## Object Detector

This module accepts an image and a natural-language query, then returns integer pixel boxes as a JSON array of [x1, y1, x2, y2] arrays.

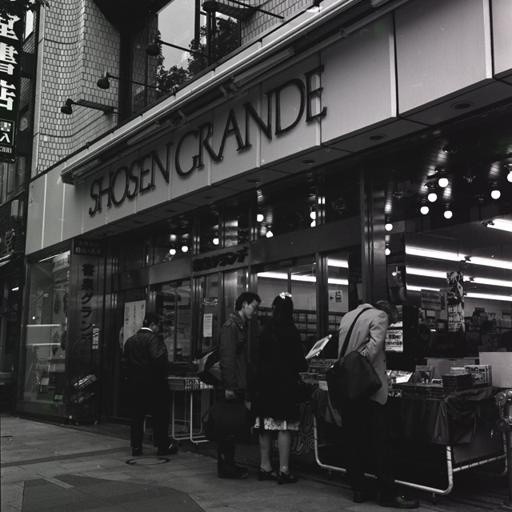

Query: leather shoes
[[379, 495, 419, 508], [354, 491, 373, 502]]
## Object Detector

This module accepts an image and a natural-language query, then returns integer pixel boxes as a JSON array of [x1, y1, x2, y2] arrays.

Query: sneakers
[[218, 463, 248, 479], [158, 447, 177, 455], [131, 447, 142, 456]]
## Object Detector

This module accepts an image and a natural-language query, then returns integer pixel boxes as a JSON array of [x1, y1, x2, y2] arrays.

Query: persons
[[336, 299, 420, 508], [253, 292, 308, 485], [124, 313, 178, 456], [213, 292, 261, 480]]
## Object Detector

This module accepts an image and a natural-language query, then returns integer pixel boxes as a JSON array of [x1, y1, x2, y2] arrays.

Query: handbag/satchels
[[326, 351, 382, 408]]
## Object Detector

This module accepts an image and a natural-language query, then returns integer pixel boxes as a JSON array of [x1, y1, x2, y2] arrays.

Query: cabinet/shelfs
[[254, 307, 349, 353]]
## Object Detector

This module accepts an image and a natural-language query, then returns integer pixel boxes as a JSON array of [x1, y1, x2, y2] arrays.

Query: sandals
[[278, 470, 297, 484], [259, 467, 278, 480]]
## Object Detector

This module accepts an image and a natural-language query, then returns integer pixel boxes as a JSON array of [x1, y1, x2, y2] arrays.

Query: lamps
[[146, 38, 209, 57], [97, 72, 170, 94], [202, 0, 284, 20], [61, 98, 117, 116]]
